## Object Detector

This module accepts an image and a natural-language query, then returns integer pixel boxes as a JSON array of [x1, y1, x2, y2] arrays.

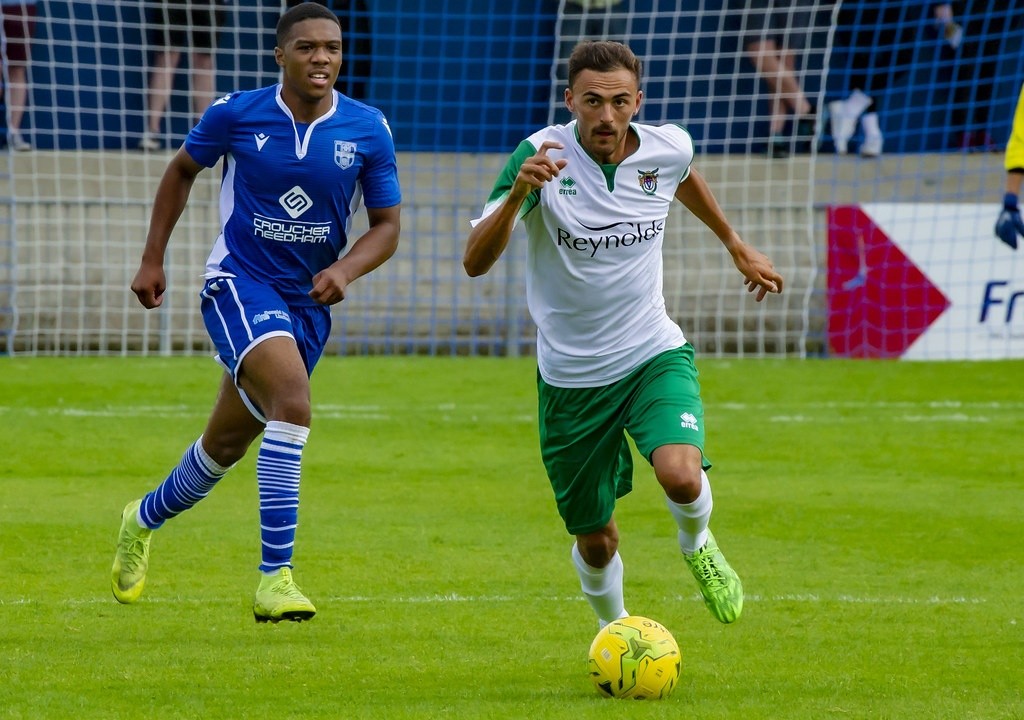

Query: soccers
[[587, 614, 682, 701]]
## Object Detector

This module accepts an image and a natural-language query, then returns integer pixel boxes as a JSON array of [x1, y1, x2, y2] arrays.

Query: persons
[[100, 5, 404, 618], [137, 1, 224, 152], [826, 0, 967, 157], [0, 0, 39, 153], [995, 81, 1024, 252], [463, 40, 784, 635], [739, 0, 813, 155]]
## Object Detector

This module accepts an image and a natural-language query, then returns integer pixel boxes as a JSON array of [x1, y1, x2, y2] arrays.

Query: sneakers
[[253, 565, 316, 621], [682, 535, 746, 624], [8, 131, 30, 151], [830, 100, 857, 154], [138, 136, 161, 151], [109, 499, 153, 604], [859, 131, 884, 157]]
